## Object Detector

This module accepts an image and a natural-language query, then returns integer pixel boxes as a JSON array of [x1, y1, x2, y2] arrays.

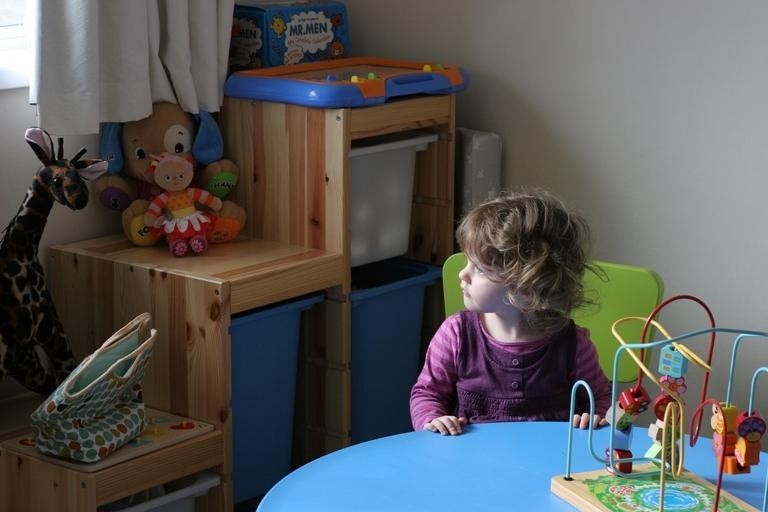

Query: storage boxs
[[114, 473, 221, 512], [348, 129, 438, 267], [229, 4, 349, 74], [227, 293, 333, 504], [350, 255, 443, 446]]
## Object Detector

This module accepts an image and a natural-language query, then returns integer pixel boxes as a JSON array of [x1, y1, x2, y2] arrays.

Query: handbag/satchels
[[28, 313, 160, 463]]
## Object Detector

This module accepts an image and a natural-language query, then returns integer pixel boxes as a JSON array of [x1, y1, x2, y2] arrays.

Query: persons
[[409, 184, 612, 436]]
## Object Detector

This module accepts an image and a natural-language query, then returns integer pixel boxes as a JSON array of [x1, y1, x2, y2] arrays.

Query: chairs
[[440, 252, 663, 385]]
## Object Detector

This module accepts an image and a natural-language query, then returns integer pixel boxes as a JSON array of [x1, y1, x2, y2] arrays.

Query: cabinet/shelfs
[[0, 92, 455, 512]]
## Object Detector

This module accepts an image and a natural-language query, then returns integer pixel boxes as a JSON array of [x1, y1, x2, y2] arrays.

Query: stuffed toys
[[94, 103, 248, 248], [0, 127, 111, 397], [144, 150, 225, 259]]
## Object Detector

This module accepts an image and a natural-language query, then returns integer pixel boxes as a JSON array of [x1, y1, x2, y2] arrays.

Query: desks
[[256, 420, 767, 512]]
